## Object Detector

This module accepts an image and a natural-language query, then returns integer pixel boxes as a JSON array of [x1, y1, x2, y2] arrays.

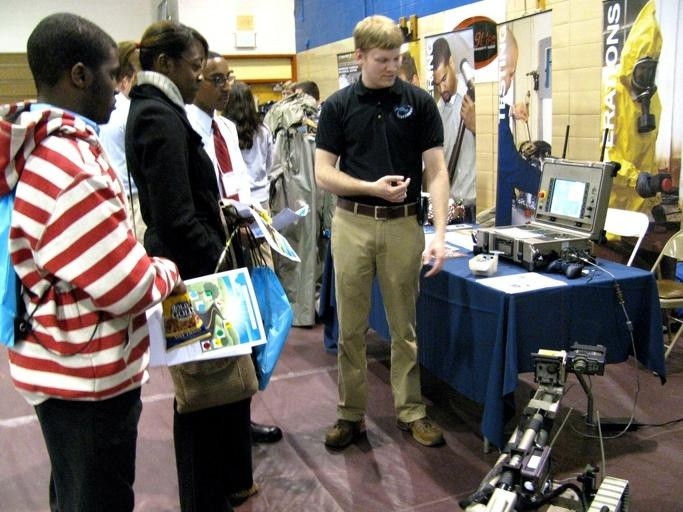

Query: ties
[[211, 118, 241, 201]]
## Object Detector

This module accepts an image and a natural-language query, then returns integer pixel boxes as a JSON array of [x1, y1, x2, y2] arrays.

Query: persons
[[496, 32, 546, 226], [221, 80, 280, 278], [402, 52, 424, 87], [277, 82, 294, 97], [104, 38, 153, 251], [0, 11, 183, 507], [313, 13, 445, 453], [116, 22, 259, 504], [289, 83, 323, 112], [431, 42, 478, 205], [602, 1, 665, 213], [187, 50, 286, 441]]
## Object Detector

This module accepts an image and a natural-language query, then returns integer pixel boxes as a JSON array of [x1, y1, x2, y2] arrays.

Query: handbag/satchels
[[127, 160, 259, 414]]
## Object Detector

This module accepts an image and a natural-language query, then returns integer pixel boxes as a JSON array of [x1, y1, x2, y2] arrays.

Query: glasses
[[205, 71, 235, 88]]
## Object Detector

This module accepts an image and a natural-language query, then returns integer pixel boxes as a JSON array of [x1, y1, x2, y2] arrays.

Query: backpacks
[[1, 135, 63, 353]]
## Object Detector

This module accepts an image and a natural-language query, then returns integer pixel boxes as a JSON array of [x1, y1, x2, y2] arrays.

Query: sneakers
[[325, 418, 366, 450], [397, 415, 443, 446]]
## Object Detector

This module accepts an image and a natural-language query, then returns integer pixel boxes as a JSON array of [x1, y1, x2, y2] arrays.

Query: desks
[[335, 219, 665, 455]]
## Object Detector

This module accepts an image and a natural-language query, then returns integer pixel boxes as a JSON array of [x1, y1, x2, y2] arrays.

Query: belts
[[337, 196, 422, 220]]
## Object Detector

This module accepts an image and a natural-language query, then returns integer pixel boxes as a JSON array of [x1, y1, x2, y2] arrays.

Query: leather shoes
[[247, 416, 283, 445]]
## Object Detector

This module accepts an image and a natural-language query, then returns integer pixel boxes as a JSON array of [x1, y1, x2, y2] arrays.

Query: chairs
[[650, 233, 682, 368]]
